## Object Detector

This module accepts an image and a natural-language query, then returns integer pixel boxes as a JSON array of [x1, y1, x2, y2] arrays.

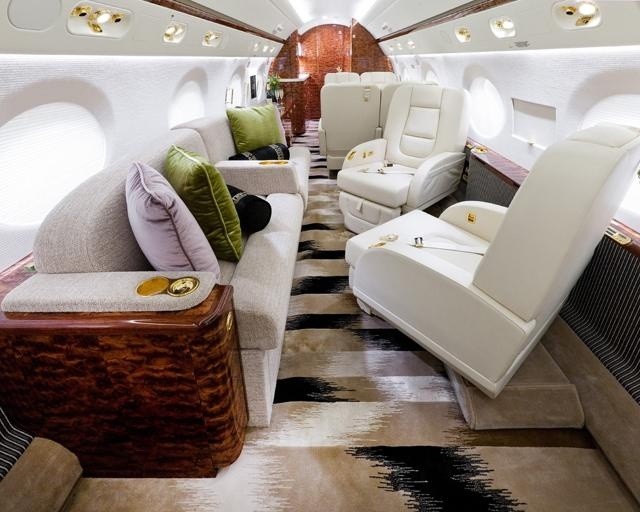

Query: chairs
[[316, 69, 439, 177], [337, 84, 472, 232], [343, 122, 639, 401]]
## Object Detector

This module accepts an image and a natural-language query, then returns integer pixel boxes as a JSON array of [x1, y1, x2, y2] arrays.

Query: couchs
[[170, 105, 311, 212], [0, 130, 304, 479]]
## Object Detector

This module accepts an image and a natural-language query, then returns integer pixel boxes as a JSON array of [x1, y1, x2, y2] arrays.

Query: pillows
[[225, 104, 290, 160], [124, 144, 271, 283]]
